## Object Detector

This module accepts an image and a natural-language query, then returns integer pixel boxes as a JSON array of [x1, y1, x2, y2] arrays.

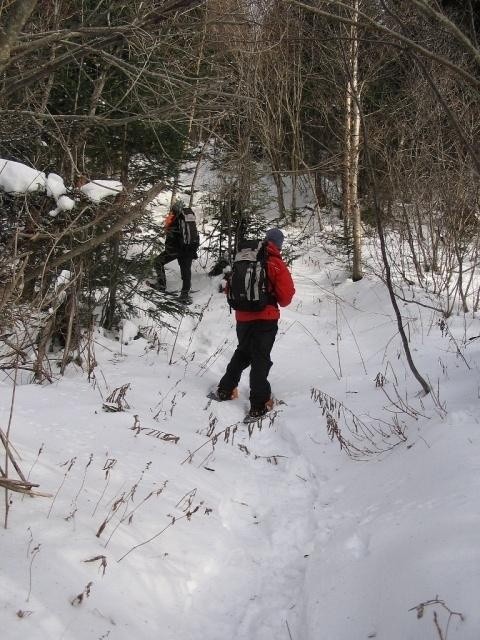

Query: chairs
[[154, 280, 166, 289], [215, 388, 239, 401], [249, 399, 273, 417], [178, 293, 188, 302]]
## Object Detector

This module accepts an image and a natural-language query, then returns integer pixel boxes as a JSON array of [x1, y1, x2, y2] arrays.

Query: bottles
[[172, 200, 186, 209], [264, 228, 285, 251]]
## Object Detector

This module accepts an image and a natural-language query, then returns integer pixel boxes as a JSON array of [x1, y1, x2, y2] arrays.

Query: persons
[[216, 227, 296, 417], [151, 198, 196, 305]]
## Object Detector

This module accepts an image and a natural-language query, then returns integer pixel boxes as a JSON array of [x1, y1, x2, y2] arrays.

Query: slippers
[[170, 207, 201, 255], [226, 238, 278, 314]]
